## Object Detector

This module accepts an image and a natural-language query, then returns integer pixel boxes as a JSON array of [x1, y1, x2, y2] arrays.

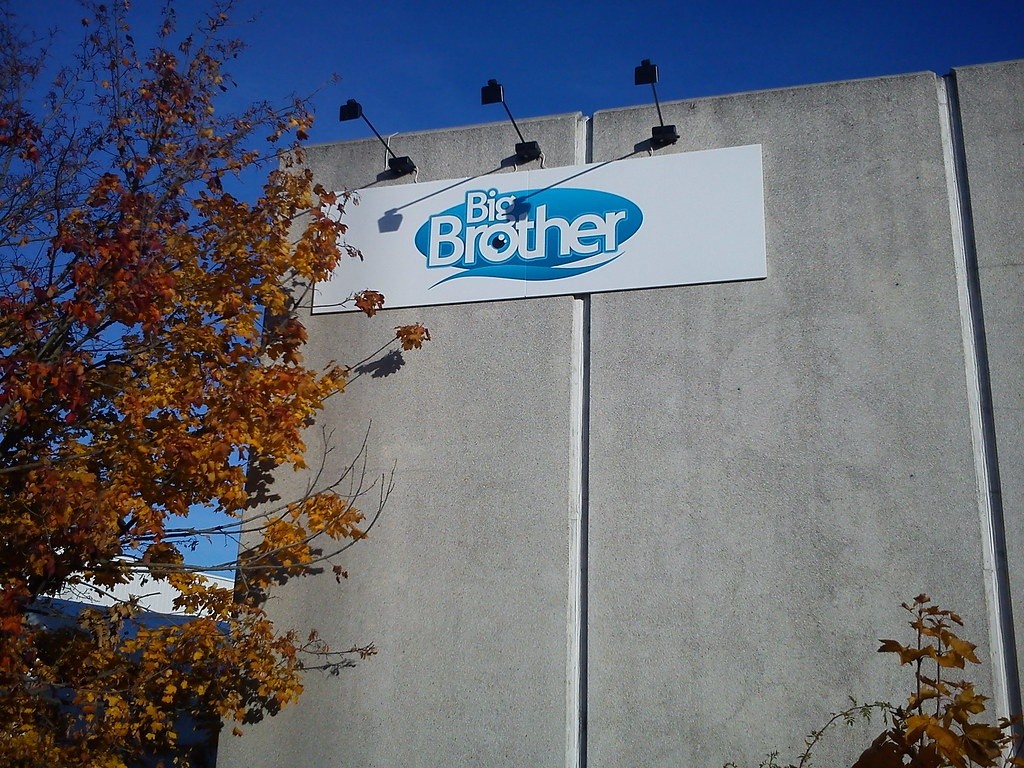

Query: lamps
[[635, 59, 677, 144], [480, 79, 542, 160], [339, 99, 416, 176]]
